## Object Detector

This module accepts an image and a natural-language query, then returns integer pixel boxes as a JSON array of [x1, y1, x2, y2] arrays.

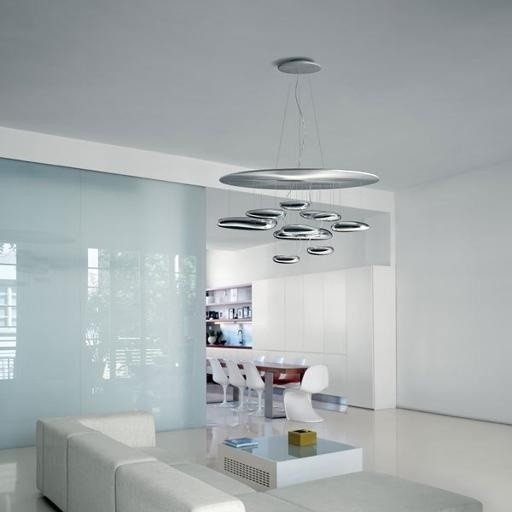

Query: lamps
[[212, 59, 383, 266]]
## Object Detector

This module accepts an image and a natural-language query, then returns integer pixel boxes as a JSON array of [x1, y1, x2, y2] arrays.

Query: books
[[223, 437, 260, 448]]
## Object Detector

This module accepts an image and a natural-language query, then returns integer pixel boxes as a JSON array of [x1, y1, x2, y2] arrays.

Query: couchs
[[33, 411, 485, 512]]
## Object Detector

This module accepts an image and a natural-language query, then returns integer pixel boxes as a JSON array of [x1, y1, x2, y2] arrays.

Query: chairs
[[205, 355, 271, 418], [281, 363, 334, 429]]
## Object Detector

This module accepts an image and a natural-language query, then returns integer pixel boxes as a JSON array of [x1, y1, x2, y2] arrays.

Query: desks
[[207, 359, 311, 420]]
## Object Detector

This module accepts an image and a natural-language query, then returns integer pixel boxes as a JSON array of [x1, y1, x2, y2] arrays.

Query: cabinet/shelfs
[[203, 280, 254, 350]]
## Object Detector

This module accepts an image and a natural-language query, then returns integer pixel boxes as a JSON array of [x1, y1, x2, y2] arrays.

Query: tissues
[[288, 428, 317, 447], [288, 445, 317, 457]]
